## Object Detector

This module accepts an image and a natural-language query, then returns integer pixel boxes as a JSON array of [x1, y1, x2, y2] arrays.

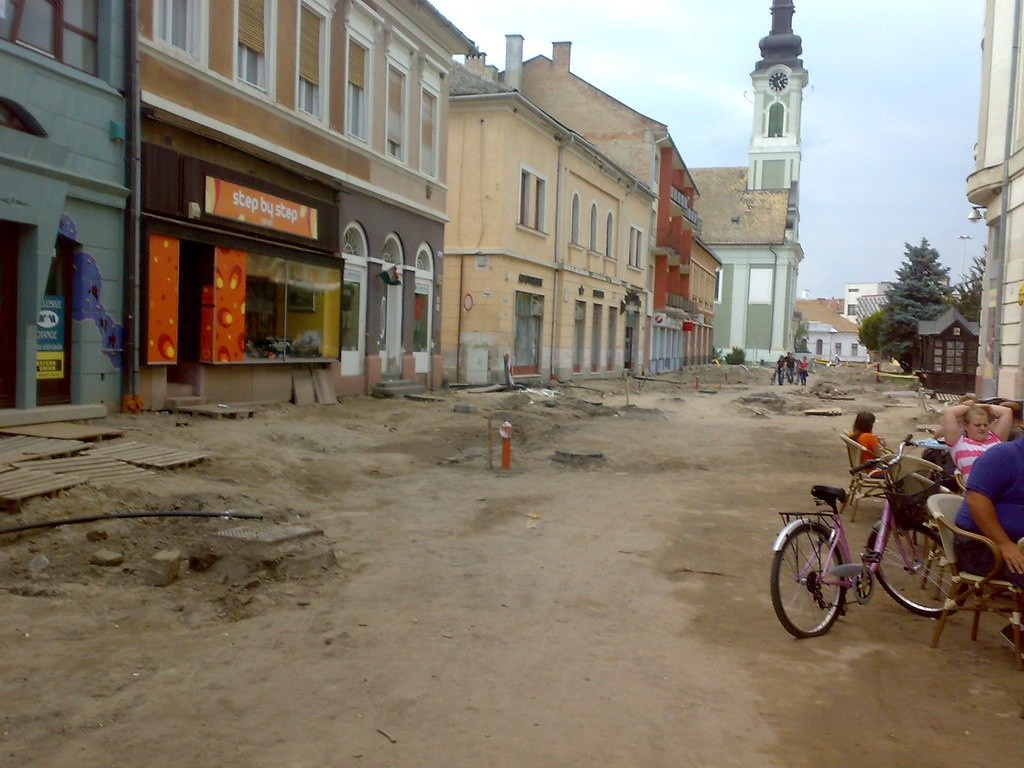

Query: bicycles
[[771, 434, 967, 640]]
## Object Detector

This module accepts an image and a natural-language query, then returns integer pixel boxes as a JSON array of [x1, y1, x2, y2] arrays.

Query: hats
[[996, 402, 1023, 427]]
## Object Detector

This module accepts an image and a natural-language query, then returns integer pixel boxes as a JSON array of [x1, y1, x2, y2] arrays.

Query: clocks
[[768, 72, 789, 91]]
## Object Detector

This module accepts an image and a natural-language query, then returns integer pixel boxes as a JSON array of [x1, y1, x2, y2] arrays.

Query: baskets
[[884, 469, 943, 531]]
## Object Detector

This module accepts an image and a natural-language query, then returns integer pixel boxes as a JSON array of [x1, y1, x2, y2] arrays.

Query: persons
[[775, 352, 809, 386], [952, 432, 1024, 658], [923, 394, 1024, 495], [847, 412, 888, 479]]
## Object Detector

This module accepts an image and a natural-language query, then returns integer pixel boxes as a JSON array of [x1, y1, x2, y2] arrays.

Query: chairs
[[839, 428, 1024, 672]]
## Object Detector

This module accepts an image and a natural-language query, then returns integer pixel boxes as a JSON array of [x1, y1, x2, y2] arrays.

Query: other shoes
[[999, 624, 1024, 660]]
